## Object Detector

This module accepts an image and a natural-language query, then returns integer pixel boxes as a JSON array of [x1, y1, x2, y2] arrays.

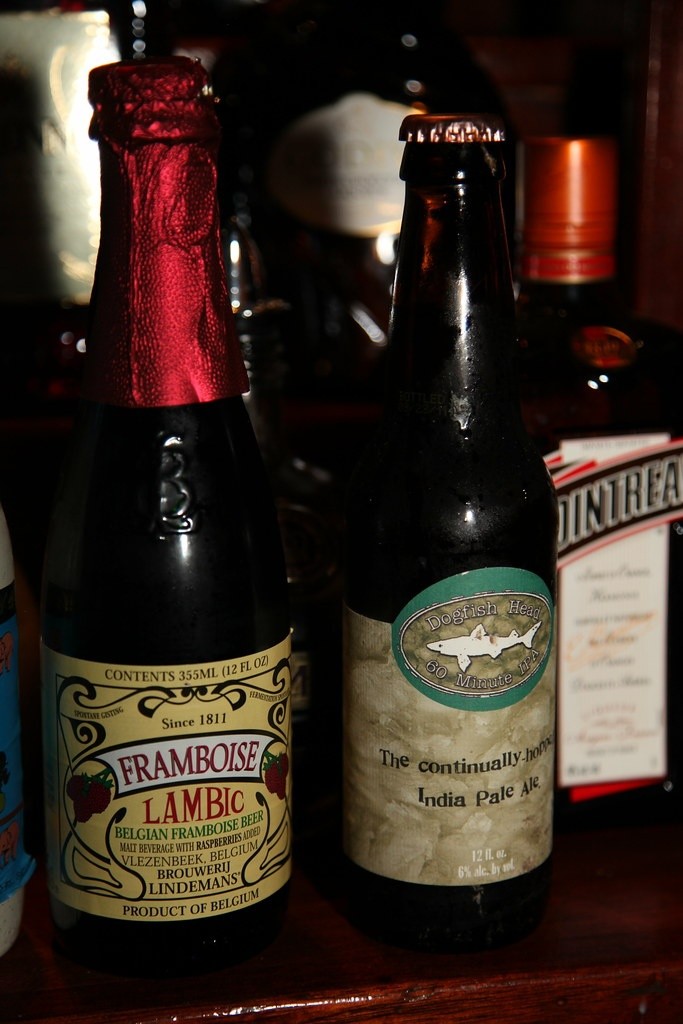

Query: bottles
[[0, 0, 683, 958]]
